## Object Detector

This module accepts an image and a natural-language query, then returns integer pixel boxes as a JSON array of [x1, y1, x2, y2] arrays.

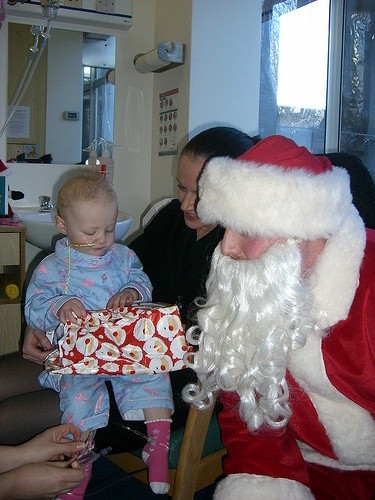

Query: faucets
[[40, 195, 54, 212]]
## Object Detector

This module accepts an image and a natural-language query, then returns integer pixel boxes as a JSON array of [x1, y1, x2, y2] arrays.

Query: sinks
[[21, 205, 134, 251]]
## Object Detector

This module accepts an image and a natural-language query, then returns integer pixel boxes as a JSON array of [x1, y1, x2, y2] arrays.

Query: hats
[[196, 135, 353, 241]]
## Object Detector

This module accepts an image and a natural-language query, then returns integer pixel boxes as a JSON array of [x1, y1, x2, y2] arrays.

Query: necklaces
[[201, 228, 211, 231]]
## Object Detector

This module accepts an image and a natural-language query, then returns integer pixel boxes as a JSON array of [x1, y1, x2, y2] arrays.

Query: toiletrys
[[96, 137, 127, 185], [82, 138, 97, 165]]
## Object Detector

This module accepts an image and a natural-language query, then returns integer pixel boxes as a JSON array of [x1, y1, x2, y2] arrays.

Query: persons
[[24, 174, 174, 500], [181, 135, 375, 500], [0, 126, 262, 443], [0, 423, 86, 500]]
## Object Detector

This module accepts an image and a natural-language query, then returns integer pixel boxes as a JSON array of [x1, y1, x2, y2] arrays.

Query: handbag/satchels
[[42, 302, 191, 375]]
